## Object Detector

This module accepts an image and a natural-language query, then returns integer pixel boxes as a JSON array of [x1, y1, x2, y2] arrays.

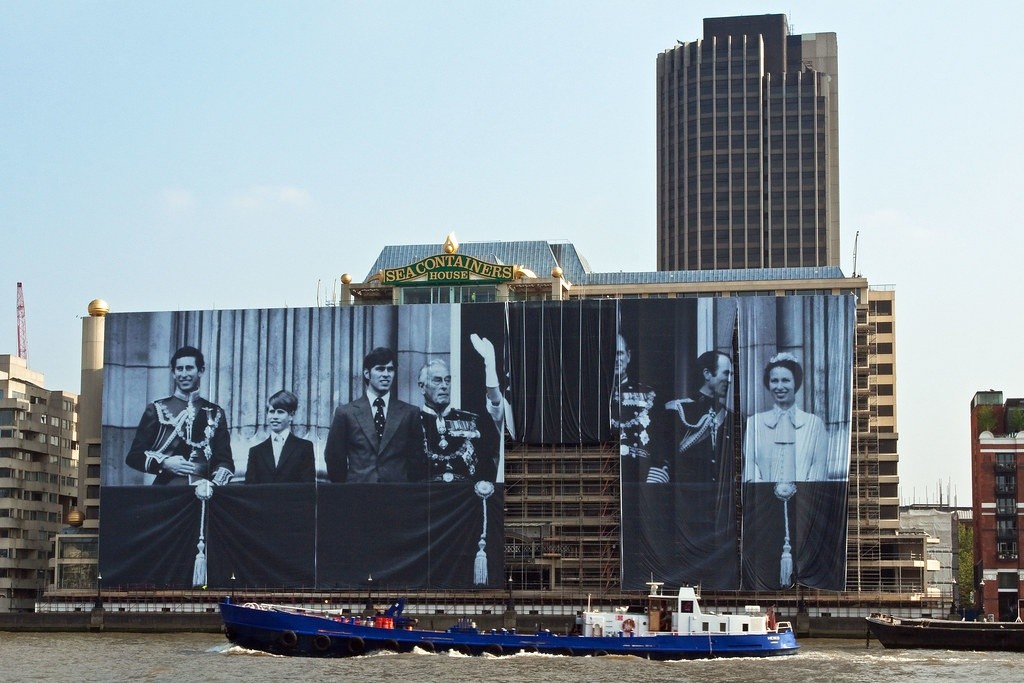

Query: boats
[[218, 571, 802, 658], [865, 609, 1024, 652]]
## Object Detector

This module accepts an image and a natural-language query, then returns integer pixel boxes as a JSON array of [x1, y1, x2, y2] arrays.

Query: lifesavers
[[348, 636, 366, 655], [280, 630, 298, 646], [379, 638, 610, 656], [623, 619, 636, 633], [313, 634, 330, 652]]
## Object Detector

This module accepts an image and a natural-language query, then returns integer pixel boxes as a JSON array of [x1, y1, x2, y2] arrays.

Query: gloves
[[470, 332, 501, 388]]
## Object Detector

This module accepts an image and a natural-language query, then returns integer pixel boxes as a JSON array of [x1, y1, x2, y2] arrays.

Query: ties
[[274, 434, 285, 465], [372, 397, 386, 440]]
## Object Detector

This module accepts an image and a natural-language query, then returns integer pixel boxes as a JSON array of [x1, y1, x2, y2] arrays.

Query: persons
[[244, 390, 316, 484], [125, 346, 236, 485], [324, 334, 516, 484], [611, 335, 828, 483]]
